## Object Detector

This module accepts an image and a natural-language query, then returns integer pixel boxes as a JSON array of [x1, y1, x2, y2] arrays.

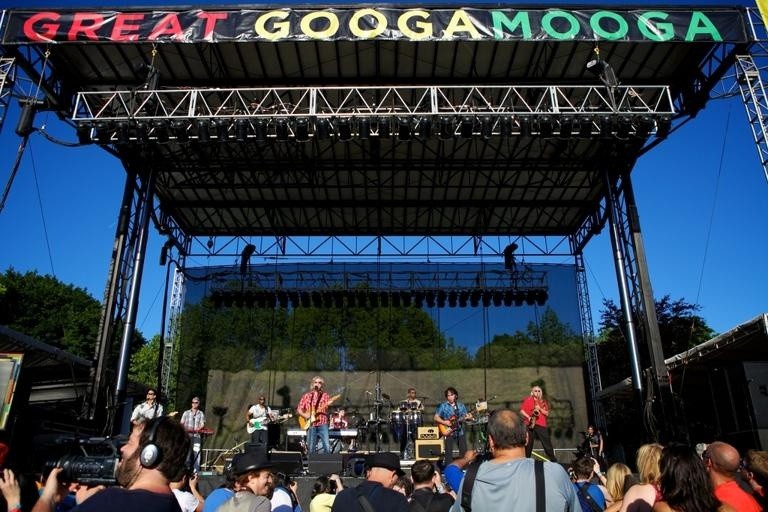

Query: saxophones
[[528, 393, 540, 429]]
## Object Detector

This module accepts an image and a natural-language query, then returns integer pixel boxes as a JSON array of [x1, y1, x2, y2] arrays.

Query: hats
[[231, 450, 274, 475], [368, 452, 406, 477]]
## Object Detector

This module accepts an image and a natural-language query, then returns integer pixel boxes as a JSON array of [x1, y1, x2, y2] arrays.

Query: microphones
[[262, 404, 267, 409], [311, 386, 318, 392], [454, 398, 458, 409]]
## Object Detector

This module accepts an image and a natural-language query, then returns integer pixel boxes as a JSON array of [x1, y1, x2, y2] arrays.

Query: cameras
[[185, 472, 195, 485]]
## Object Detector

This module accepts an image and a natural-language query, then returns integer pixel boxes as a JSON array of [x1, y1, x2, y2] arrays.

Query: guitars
[[438, 412, 473, 435], [247, 414, 292, 434], [298, 394, 341, 429]]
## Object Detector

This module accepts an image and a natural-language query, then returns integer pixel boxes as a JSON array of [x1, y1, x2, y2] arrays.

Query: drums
[[404, 411, 421, 424], [392, 411, 403, 422], [400, 401, 408, 411], [410, 402, 417, 410]]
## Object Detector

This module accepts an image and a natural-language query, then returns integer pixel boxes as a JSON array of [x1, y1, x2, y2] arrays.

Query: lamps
[[582, 43, 617, 89], [502, 242, 518, 270], [238, 244, 255, 274], [15, 96, 47, 137], [201, 286, 553, 310], [158, 237, 177, 266]]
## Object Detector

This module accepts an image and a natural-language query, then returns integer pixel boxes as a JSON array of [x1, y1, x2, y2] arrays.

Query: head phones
[[139, 416, 170, 469]]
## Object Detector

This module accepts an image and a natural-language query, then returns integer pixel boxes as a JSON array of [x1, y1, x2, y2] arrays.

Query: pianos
[[286, 429, 358, 437]]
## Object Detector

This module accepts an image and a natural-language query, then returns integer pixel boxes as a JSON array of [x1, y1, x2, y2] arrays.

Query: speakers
[[75, 416, 105, 437], [343, 453, 373, 477], [415, 439, 445, 461], [269, 453, 303, 477], [308, 454, 344, 477], [245, 441, 267, 459]]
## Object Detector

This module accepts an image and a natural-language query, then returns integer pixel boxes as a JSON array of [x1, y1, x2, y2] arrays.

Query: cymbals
[[416, 397, 429, 399]]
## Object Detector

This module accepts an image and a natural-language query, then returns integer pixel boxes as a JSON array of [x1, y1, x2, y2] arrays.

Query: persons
[[518, 385, 556, 462], [297, 375, 333, 453], [179, 396, 208, 473], [129, 388, 164, 426], [1, 408, 767, 512], [245, 396, 275, 446], [433, 387, 472, 467], [398, 387, 425, 458]]
